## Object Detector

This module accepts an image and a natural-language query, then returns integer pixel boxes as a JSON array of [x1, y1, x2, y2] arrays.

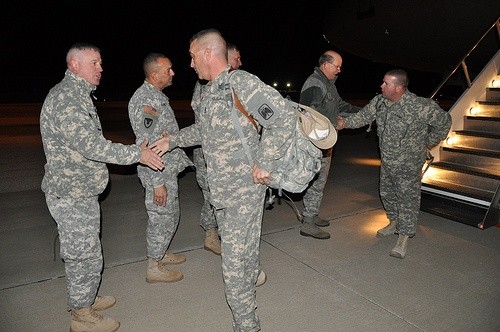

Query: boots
[[162, 250, 187, 265], [204, 228, 221, 255], [389, 232, 409, 259], [89, 295, 116, 311], [69, 306, 121, 332], [255, 270, 267, 287], [314, 215, 330, 227], [299, 217, 331, 239], [377, 218, 399, 237], [145, 258, 183, 284]]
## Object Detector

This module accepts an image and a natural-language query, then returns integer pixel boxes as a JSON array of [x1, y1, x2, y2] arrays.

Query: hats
[[297, 102, 338, 150]]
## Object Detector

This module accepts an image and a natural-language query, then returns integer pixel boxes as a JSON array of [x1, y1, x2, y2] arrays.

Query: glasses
[[326, 60, 342, 69]]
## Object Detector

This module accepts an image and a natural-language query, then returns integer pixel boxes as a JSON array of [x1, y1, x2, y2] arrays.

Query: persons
[[148, 29, 294, 332], [301, 51, 372, 239], [192, 45, 242, 255], [128, 53, 193, 283], [336, 69, 453, 257], [40, 43, 166, 332]]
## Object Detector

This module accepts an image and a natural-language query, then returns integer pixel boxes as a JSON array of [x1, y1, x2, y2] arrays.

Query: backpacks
[[226, 68, 324, 195]]
